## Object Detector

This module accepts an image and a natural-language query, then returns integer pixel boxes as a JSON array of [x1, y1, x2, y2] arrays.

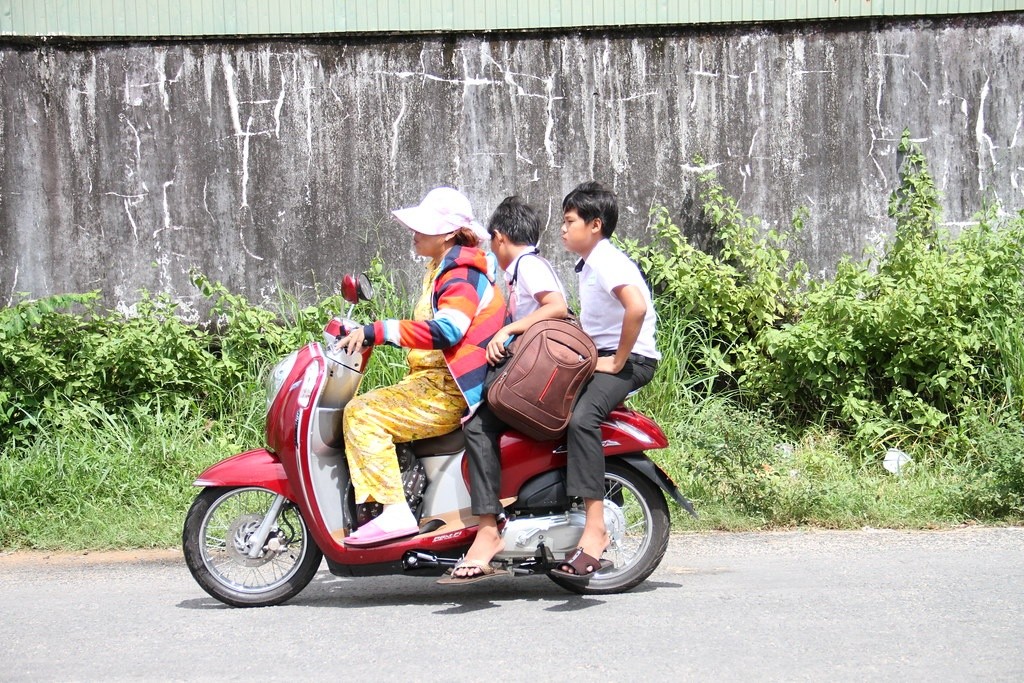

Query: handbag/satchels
[[488, 255, 598, 443]]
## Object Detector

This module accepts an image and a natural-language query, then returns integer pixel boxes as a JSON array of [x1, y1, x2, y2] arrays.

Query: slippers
[[342, 520, 419, 545], [550, 547, 614, 580], [436, 560, 512, 585]]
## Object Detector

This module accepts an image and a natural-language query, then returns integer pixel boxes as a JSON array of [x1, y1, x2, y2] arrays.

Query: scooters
[[183, 274, 697, 608]]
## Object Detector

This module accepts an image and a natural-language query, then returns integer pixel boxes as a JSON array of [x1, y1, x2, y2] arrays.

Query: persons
[[334, 180, 662, 585]]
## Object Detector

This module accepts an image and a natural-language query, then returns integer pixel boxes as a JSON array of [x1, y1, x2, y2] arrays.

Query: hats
[[392, 187, 492, 240]]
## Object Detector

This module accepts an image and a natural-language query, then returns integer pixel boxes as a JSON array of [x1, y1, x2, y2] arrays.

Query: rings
[[349, 335, 353, 338]]
